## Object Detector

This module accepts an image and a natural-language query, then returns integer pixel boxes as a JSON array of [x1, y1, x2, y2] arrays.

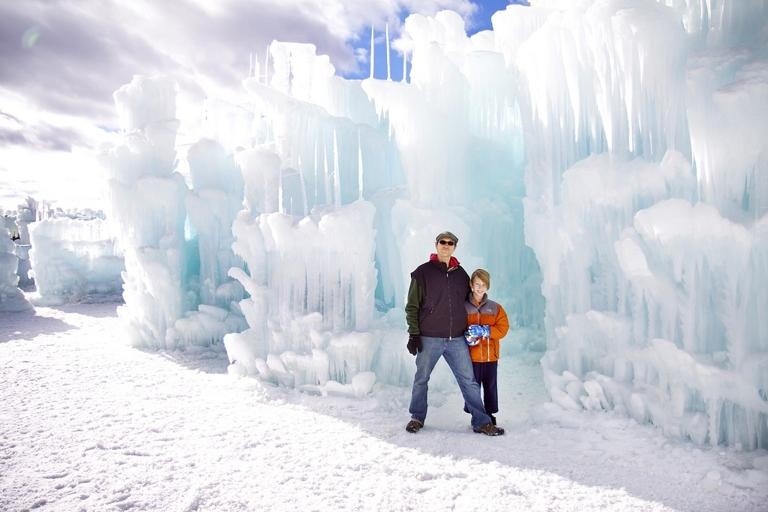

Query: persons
[[405, 232, 509, 436]]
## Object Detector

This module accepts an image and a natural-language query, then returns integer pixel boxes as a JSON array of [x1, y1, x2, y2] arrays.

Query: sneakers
[[406, 420, 424, 432], [473, 424, 504, 436]]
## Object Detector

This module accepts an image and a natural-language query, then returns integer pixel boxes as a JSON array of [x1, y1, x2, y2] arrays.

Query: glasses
[[439, 240, 456, 246]]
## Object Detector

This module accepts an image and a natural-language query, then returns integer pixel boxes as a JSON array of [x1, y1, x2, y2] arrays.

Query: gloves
[[407, 334, 422, 355], [463, 332, 480, 347], [469, 324, 490, 339]]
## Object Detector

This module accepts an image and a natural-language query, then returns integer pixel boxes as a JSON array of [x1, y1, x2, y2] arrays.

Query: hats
[[435, 231, 457, 243]]
